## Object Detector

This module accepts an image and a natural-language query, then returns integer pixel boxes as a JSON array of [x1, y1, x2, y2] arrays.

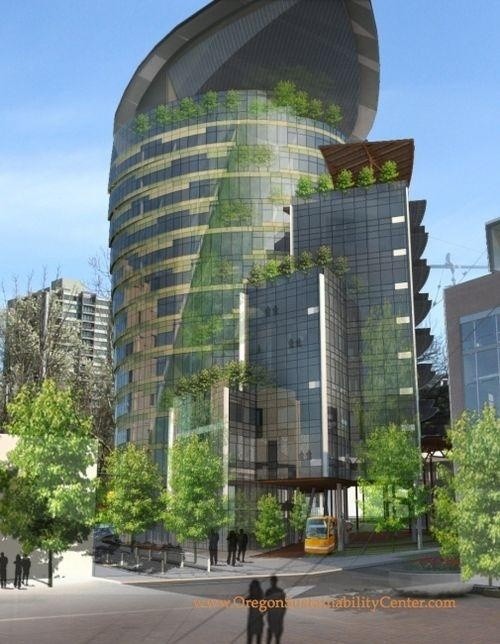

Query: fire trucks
[[302, 516, 338, 555]]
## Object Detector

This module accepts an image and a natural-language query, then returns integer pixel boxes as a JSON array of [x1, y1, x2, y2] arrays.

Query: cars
[[341, 518, 354, 532], [93, 527, 185, 572]]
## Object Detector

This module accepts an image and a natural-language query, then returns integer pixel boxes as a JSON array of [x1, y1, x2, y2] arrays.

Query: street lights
[[421, 448, 445, 540]]
